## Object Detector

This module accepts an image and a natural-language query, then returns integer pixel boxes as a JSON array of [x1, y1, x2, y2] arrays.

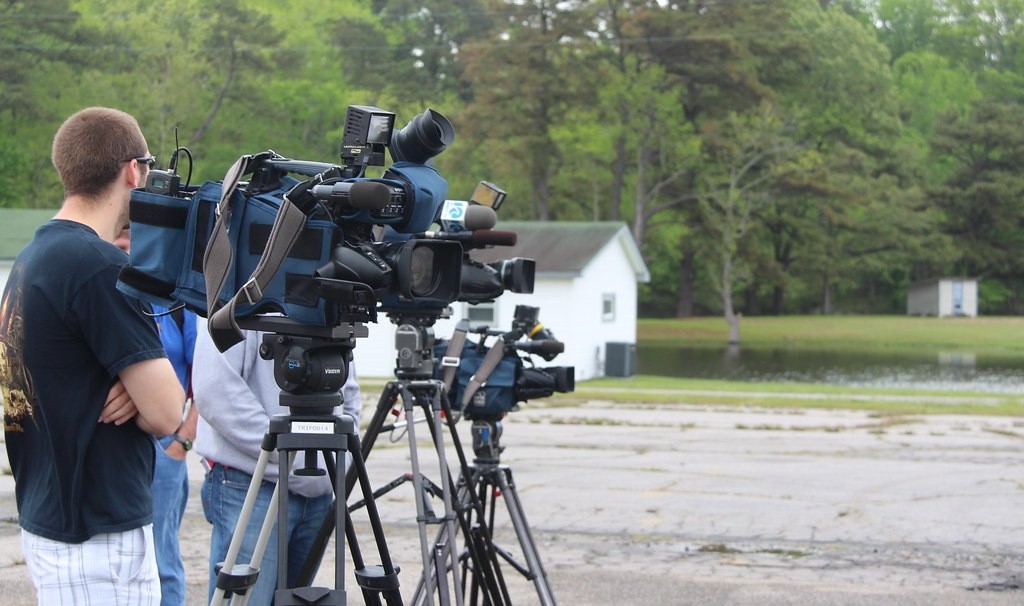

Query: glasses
[[121, 155, 156, 170]]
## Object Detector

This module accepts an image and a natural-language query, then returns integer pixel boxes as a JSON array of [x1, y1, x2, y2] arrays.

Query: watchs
[[175, 435, 193, 451]]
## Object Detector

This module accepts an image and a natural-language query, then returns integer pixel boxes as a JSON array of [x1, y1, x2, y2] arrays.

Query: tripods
[[209, 316, 556, 606]]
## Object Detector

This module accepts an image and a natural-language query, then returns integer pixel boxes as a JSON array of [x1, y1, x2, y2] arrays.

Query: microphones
[[311, 183, 390, 211], [433, 199, 517, 252], [515, 339, 565, 354]]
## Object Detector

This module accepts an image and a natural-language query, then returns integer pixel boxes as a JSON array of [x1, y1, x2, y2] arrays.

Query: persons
[[190, 311, 363, 606], [0, 106, 187, 605], [112, 228, 197, 606]]
